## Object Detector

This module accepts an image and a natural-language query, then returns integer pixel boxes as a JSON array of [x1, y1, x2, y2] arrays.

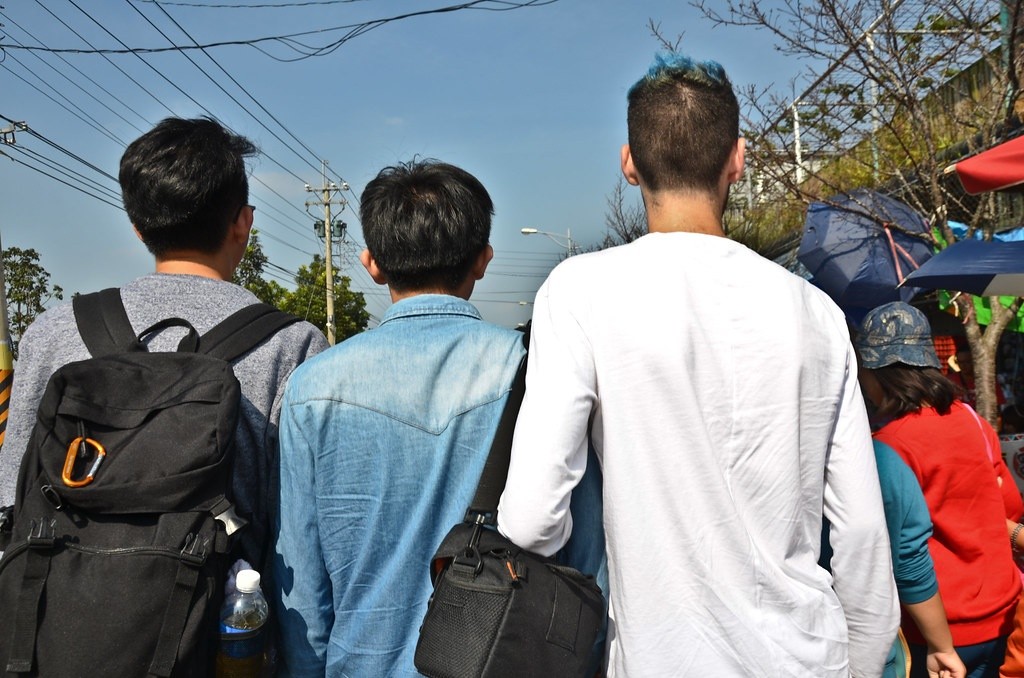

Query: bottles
[[219, 570, 268, 636]]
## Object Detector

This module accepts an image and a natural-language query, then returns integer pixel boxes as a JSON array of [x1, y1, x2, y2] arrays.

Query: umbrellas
[[893, 212, 1024, 350], [797, 186, 934, 322]]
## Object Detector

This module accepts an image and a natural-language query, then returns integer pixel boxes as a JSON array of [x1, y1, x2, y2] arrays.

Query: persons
[[1000, 403, 1024, 435], [850, 302, 1024, 678], [260, 152, 604, 678], [994, 476, 1024, 678], [495, 56, 901, 678], [0, 116, 335, 678], [804, 432, 973, 678], [942, 345, 1005, 435]]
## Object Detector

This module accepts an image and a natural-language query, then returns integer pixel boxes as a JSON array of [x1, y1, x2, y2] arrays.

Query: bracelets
[[1010, 523, 1024, 553]]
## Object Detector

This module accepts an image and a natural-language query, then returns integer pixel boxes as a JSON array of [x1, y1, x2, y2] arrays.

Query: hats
[[854, 301, 943, 369]]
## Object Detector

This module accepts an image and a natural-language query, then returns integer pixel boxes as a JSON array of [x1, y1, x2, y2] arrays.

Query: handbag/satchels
[[412, 354, 609, 678]]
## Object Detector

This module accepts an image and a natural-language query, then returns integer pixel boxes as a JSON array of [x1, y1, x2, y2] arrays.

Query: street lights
[[521, 227, 572, 259]]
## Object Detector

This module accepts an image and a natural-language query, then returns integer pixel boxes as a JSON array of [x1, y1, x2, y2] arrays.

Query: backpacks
[[0, 287, 304, 678]]
